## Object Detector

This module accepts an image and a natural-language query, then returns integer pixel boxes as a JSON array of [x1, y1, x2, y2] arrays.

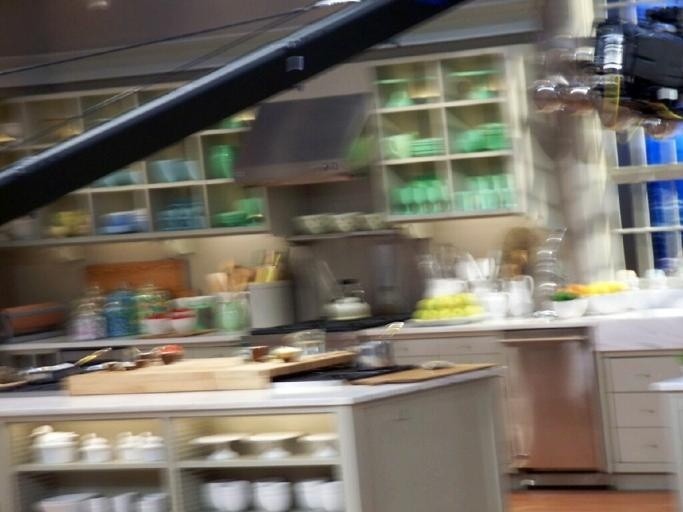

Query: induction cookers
[[247, 311, 410, 334]]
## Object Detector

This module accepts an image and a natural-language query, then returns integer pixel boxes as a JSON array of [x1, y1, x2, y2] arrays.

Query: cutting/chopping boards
[[348, 362, 499, 384], [56, 348, 358, 398]]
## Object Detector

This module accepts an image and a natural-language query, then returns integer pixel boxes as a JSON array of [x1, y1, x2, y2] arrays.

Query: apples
[[412, 293, 480, 319]]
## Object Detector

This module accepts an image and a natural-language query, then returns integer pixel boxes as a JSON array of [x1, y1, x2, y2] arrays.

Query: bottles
[[211, 293, 242, 332], [73, 282, 175, 343], [245, 277, 296, 329]]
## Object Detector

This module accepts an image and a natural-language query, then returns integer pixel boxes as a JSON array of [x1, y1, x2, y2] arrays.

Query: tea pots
[[322, 278, 374, 323]]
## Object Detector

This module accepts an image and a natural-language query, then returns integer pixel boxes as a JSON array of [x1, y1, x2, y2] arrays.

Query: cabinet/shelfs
[[0, 44, 532, 249], [1, 368, 507, 512], [596, 349, 683, 490]]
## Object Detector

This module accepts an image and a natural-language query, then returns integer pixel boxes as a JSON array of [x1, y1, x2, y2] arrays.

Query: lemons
[[581, 280, 624, 296]]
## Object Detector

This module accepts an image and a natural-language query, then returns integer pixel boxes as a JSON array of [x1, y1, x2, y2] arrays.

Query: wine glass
[[399, 176, 451, 216]]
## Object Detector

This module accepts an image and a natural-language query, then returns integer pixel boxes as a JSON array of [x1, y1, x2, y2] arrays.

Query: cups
[[427, 277, 471, 297], [488, 274, 534, 318], [458, 173, 517, 209], [388, 132, 416, 159]]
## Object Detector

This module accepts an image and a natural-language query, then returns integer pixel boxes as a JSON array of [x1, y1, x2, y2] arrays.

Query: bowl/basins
[[203, 477, 344, 512], [142, 307, 200, 335], [92, 142, 239, 185], [20, 346, 115, 383], [303, 211, 389, 234], [585, 291, 634, 315], [554, 298, 588, 319], [187, 432, 340, 460]]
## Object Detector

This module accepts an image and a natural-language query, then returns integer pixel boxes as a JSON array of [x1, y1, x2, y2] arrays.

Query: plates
[[466, 121, 510, 152], [406, 313, 483, 330], [411, 137, 445, 156], [29, 425, 172, 512], [6, 195, 267, 240]]
